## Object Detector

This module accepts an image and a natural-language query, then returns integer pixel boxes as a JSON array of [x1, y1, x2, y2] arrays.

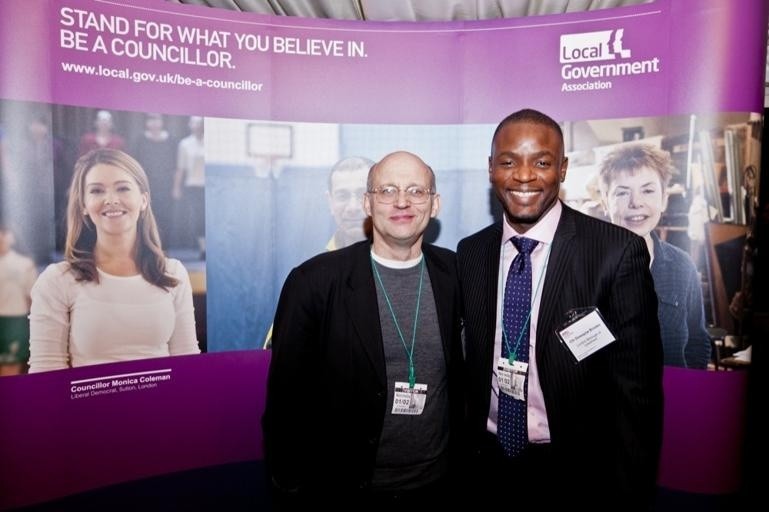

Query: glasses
[[367, 184, 435, 204]]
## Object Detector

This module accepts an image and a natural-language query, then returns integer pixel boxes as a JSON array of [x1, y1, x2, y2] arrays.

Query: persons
[[260, 150, 471, 511], [451, 106, 667, 512], [0, 220, 41, 371], [134, 112, 174, 238], [78, 109, 125, 151], [170, 116, 204, 260], [324, 155, 378, 254], [595, 142, 711, 370], [729, 290, 751, 324], [26, 149, 201, 374]]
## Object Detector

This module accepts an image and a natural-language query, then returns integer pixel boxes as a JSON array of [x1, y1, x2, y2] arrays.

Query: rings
[[733, 306, 739, 314]]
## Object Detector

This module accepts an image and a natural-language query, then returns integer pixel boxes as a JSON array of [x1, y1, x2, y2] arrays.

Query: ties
[[496, 236, 540, 461]]
